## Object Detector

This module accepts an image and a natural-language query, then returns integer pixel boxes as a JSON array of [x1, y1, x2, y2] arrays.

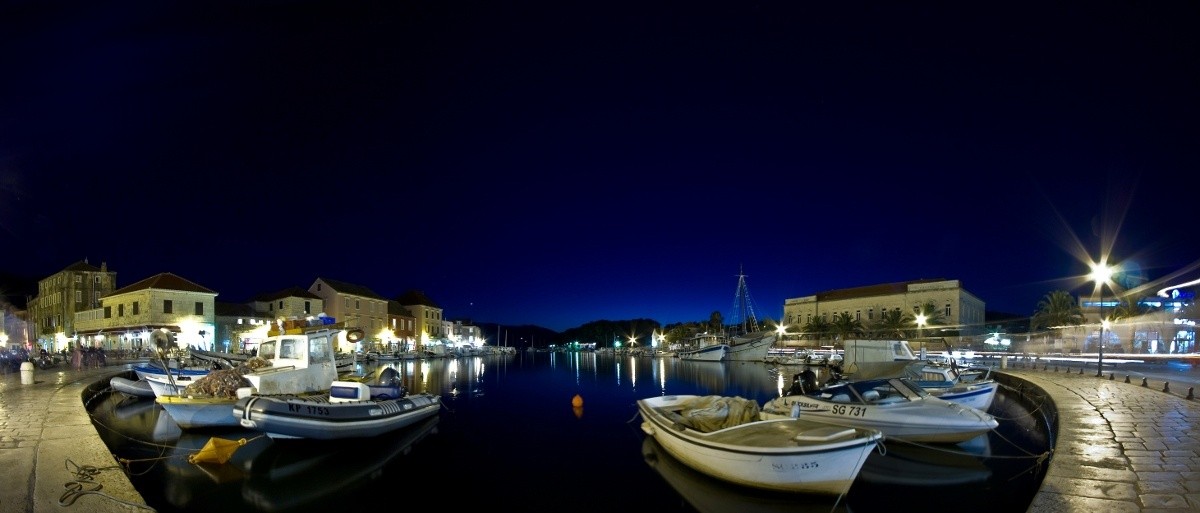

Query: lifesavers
[[347, 330, 365, 343]]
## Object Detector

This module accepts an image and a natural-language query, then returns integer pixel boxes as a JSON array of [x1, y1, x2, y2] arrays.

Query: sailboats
[[721, 262, 777, 362], [495, 324, 516, 353]]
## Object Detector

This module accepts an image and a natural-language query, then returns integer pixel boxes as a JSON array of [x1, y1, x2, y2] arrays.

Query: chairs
[[832, 394, 850, 403], [862, 390, 880, 402]]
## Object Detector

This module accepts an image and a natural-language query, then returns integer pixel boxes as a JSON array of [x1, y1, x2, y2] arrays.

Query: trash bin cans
[[19, 362, 34, 384]]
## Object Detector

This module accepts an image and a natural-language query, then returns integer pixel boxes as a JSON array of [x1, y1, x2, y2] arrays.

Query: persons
[[639, 351, 645, 358], [72, 345, 108, 372]]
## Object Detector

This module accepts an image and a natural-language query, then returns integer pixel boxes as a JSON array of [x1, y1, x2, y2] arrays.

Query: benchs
[[793, 428, 856, 446]]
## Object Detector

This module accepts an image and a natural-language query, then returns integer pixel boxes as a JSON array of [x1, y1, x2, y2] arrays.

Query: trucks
[[843, 339, 920, 364]]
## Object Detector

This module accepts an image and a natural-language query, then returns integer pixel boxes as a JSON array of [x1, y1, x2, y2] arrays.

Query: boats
[[676, 343, 729, 362], [859, 429, 996, 485], [640, 435, 854, 513], [104, 311, 444, 443], [237, 413, 442, 512], [353, 344, 495, 363], [763, 359, 1001, 445], [635, 394, 883, 498]]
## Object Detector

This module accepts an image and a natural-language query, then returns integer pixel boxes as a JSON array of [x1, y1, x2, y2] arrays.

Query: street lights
[[1096, 263, 1112, 377], [919, 317, 925, 360], [778, 325, 784, 356]]
